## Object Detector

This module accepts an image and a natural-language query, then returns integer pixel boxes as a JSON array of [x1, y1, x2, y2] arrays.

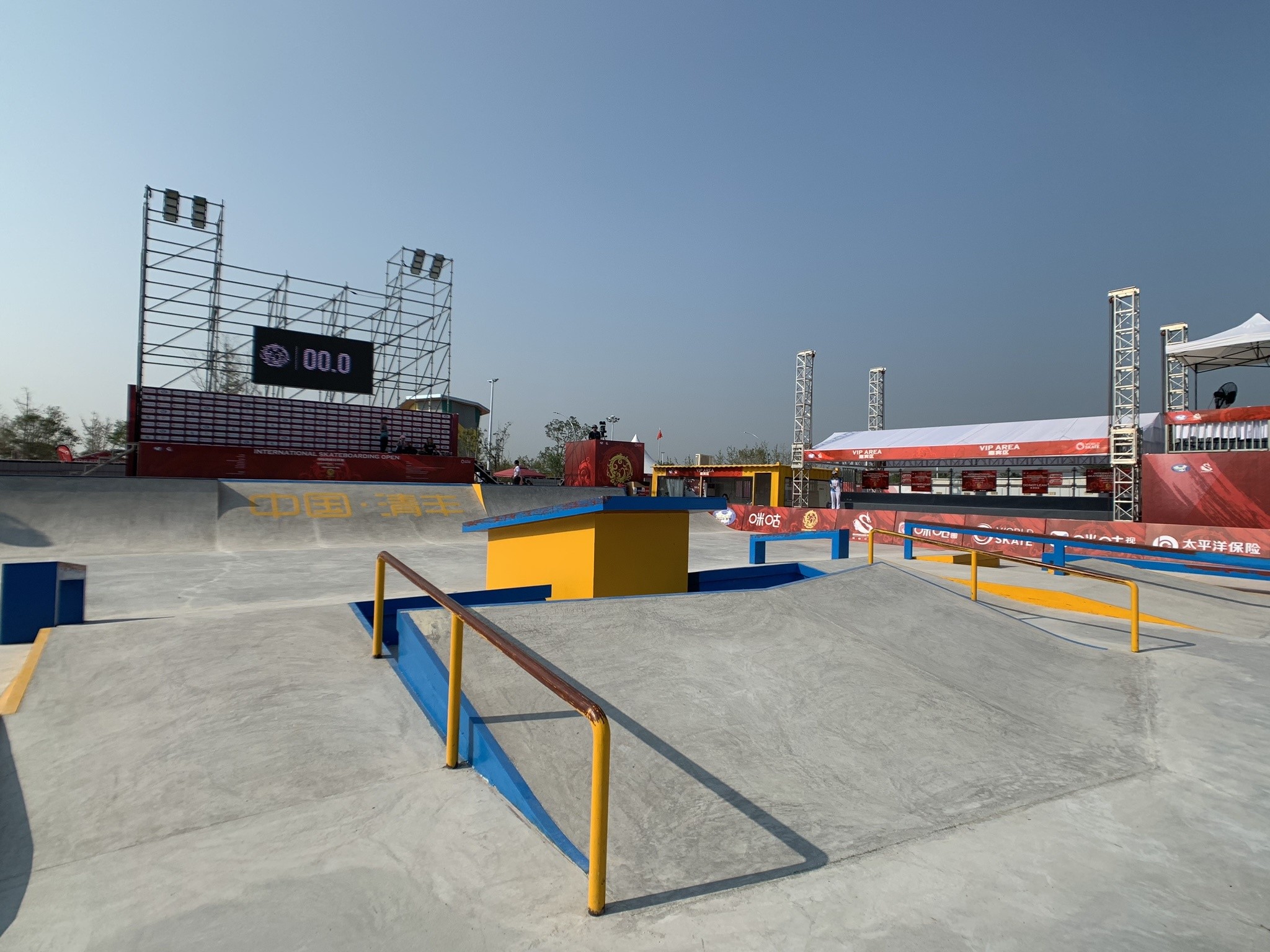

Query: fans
[[1213, 382, 1238, 409]]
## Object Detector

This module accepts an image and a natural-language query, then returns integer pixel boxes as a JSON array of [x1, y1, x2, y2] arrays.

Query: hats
[[832, 470, 838, 474], [400, 435, 406, 438], [592, 424, 598, 429]]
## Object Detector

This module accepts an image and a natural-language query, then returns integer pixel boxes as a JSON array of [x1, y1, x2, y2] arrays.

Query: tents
[[800, 413, 1165, 509], [1166, 313, 1270, 412]]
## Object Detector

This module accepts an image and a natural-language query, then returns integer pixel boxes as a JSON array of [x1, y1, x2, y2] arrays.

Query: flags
[[657, 430, 663, 439]]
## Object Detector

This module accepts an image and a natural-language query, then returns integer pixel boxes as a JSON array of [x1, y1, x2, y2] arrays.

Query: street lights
[[605, 412, 620, 440], [744, 431, 768, 464], [552, 412, 576, 441], [487, 378, 500, 470], [660, 451, 665, 465]]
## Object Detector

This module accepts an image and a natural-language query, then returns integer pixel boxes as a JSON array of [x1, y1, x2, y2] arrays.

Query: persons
[[380, 417, 389, 453], [588, 425, 600, 440], [723, 494, 730, 502], [422, 437, 437, 453], [829, 470, 843, 509], [513, 460, 521, 485], [395, 435, 406, 451]]
